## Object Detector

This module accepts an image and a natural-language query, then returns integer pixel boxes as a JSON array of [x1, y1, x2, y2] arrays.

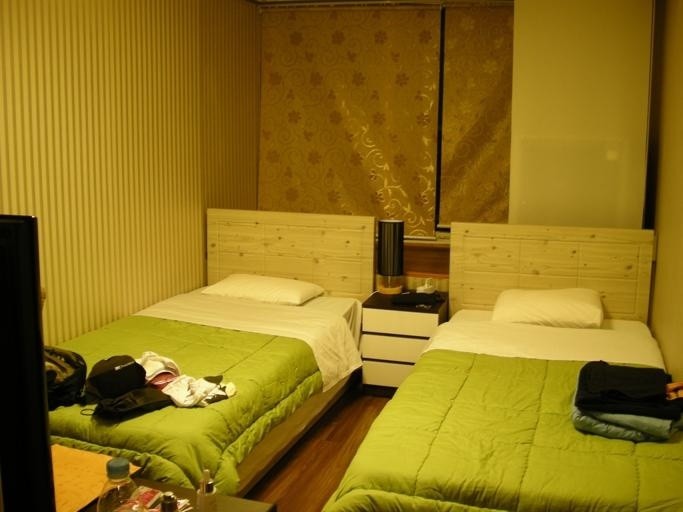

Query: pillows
[[490, 288, 604, 330], [199, 271, 324, 306]]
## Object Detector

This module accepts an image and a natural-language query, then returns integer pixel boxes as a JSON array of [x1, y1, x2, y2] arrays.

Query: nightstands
[[359, 290, 448, 392]]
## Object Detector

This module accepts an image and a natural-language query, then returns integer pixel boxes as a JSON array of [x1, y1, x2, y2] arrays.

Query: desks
[[78, 478, 275, 512]]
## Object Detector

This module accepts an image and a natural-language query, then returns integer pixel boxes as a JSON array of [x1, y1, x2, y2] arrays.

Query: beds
[[318, 220, 681, 512], [42, 208, 376, 500]]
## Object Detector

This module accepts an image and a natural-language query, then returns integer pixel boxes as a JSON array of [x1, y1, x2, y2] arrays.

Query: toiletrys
[[159, 491, 179, 512], [195, 469, 218, 512]]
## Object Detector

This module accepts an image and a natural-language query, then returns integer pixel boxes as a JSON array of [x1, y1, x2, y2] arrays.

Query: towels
[[139, 351, 181, 383]]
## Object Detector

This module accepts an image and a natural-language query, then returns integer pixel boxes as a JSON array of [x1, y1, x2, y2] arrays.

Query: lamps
[[376, 220, 404, 294]]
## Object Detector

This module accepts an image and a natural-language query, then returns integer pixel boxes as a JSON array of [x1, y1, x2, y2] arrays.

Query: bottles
[[96, 458, 142, 512]]
